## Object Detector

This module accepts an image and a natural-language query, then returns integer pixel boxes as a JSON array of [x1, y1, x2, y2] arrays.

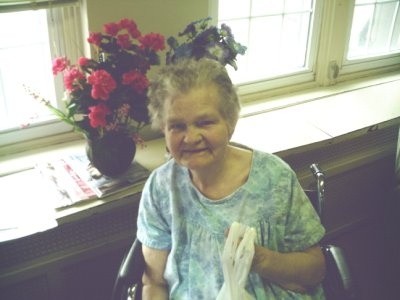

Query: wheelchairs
[[111, 163, 358, 300]]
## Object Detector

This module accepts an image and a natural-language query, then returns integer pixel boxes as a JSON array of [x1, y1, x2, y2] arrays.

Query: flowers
[[21, 17, 167, 138], [165, 16, 248, 70]]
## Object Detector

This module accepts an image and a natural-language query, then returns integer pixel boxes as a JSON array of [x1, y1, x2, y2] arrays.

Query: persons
[[136, 55, 328, 300]]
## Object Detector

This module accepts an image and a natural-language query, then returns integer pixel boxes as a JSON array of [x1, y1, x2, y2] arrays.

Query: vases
[[81, 132, 136, 175]]
